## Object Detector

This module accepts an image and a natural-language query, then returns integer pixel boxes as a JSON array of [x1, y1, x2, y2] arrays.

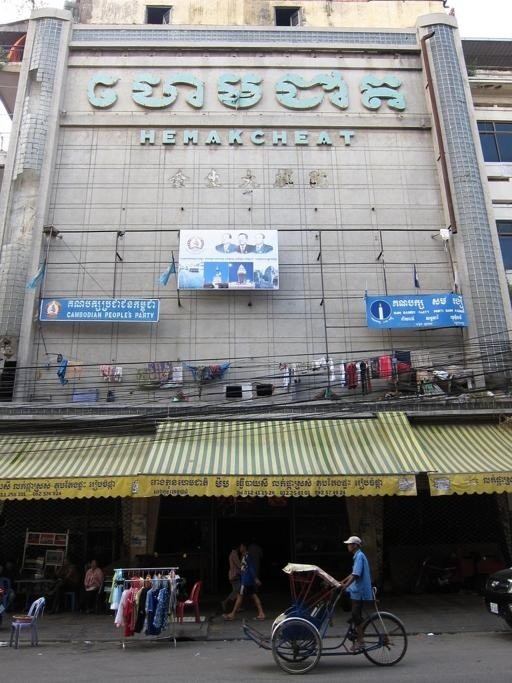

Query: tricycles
[[409, 544, 506, 594], [240, 563, 407, 675]]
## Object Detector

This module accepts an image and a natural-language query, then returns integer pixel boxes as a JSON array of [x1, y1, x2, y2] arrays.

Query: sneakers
[[350, 641, 363, 651]]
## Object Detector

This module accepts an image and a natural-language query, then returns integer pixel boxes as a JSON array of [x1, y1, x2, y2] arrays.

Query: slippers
[[220, 612, 268, 620]]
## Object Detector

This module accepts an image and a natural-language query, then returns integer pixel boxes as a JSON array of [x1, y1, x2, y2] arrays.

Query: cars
[[481, 565, 512, 630]]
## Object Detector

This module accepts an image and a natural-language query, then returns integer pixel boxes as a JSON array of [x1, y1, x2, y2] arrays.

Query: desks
[[14, 578, 55, 613]]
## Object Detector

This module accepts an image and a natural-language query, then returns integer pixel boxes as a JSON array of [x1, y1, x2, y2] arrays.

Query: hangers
[[121, 568, 173, 589]]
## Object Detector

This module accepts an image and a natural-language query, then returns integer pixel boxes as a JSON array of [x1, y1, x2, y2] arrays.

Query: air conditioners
[[223, 383, 253, 400]]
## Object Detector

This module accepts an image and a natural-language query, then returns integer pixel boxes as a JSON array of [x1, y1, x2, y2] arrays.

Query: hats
[[341, 534, 362, 546]]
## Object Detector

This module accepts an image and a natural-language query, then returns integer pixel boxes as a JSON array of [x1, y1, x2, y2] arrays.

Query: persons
[[339, 536, 374, 652], [78, 556, 104, 612], [221, 539, 267, 621], [251, 233, 275, 253], [232, 232, 254, 253], [46, 553, 74, 615], [216, 233, 238, 252], [221, 545, 244, 611]]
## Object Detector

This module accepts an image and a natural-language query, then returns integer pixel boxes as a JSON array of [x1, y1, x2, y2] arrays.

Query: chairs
[[0, 577, 10, 620], [177, 581, 201, 624], [8, 597, 46, 650]]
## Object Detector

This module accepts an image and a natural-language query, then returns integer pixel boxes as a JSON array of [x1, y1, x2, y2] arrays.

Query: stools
[[91, 591, 103, 614], [64, 592, 79, 610]]
